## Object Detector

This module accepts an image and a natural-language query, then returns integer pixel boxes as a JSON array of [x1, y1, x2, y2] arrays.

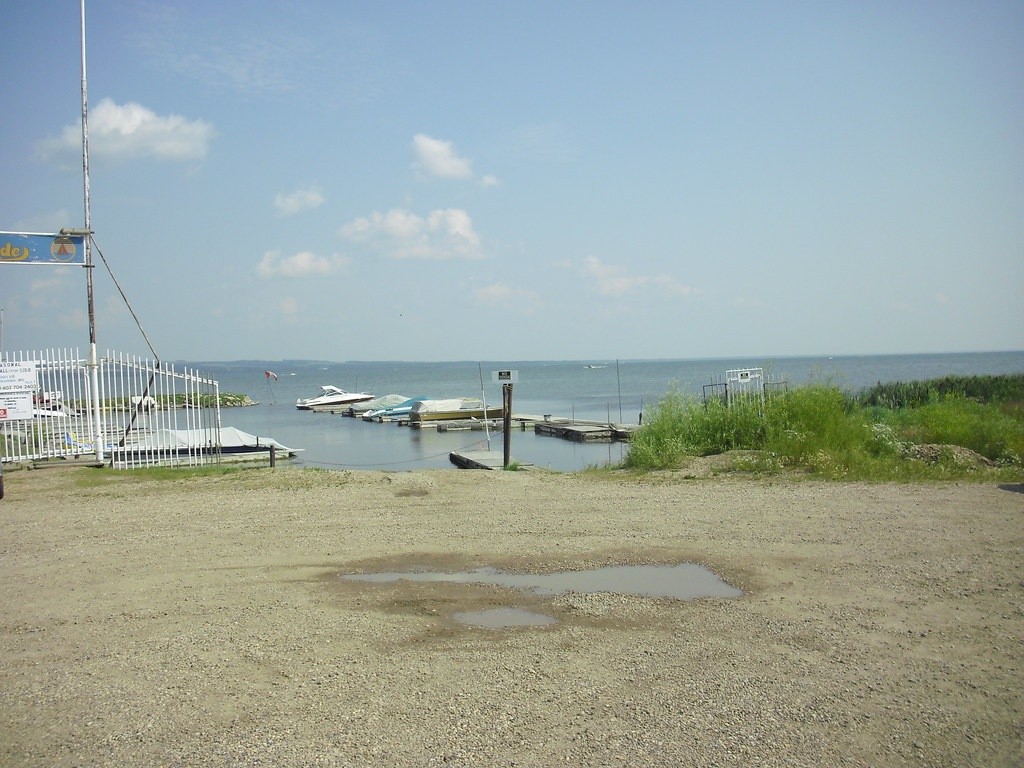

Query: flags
[[265, 371, 278, 381]]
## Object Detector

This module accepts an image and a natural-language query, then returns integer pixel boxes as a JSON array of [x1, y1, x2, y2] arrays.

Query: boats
[[295, 384, 375, 410], [342, 393, 506, 429], [131, 395, 157, 412], [101, 426, 307, 469], [609, 422, 636, 438], [33, 404, 68, 418]]
